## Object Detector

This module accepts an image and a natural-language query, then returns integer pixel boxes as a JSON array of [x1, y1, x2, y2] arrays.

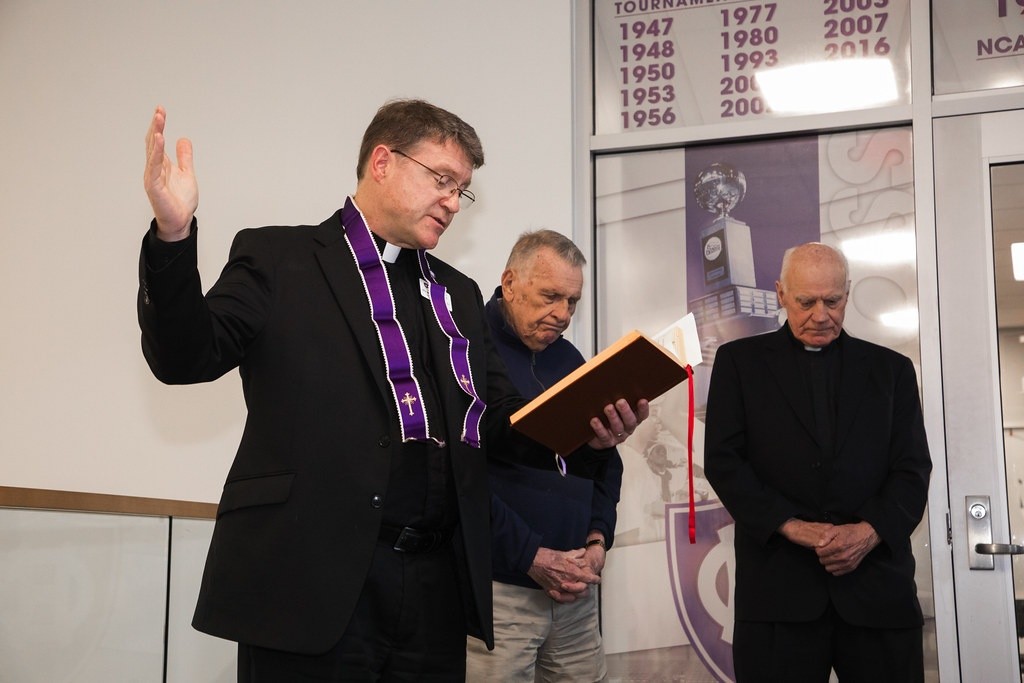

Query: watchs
[[584, 538, 606, 551]]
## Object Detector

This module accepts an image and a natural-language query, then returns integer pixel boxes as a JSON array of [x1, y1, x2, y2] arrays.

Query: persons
[[137, 99, 652, 683], [464, 231, 626, 683], [703, 243, 931, 683]]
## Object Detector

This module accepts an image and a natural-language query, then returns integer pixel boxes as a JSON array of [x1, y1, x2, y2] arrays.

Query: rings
[[612, 430, 625, 437]]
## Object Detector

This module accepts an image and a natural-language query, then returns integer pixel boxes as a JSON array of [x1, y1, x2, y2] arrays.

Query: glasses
[[390, 148, 476, 209]]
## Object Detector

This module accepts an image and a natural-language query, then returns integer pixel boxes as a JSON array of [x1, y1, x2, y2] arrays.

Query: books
[[510, 329, 696, 545]]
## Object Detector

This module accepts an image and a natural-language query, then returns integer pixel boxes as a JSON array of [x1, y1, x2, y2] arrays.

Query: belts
[[375, 519, 441, 555]]
